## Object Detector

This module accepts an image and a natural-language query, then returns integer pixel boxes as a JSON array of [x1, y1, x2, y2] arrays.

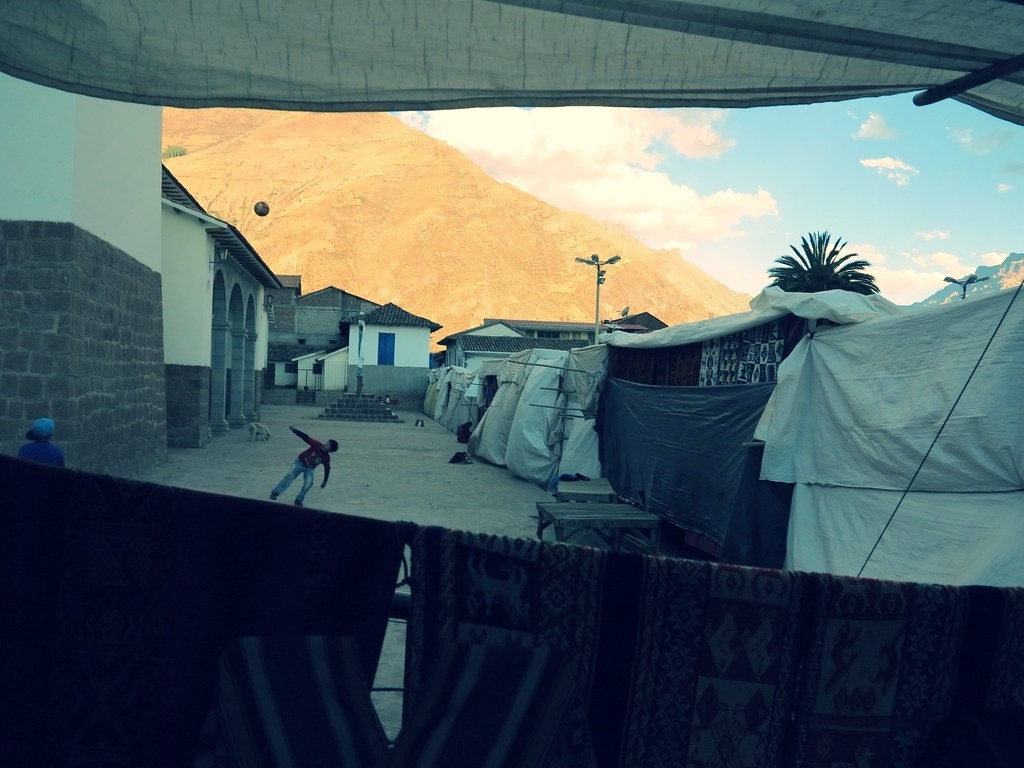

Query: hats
[[32, 418, 54, 437]]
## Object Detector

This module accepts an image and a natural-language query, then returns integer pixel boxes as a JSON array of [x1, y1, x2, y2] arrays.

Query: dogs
[[248, 423, 271, 442]]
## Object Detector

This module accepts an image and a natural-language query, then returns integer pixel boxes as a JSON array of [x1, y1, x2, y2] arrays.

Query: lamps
[[262, 296, 273, 309], [210, 248, 229, 266]]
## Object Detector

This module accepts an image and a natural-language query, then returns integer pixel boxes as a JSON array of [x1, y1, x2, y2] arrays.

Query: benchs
[[536, 500, 662, 557], [556, 478, 617, 505]]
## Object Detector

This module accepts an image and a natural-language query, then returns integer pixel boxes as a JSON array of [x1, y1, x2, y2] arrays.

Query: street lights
[[573, 253, 622, 347], [943, 273, 990, 300]]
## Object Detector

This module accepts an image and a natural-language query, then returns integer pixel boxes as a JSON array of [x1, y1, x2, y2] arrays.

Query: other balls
[[254, 202, 269, 216]]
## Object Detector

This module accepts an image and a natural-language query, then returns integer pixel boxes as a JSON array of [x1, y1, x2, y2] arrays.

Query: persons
[[268, 423, 339, 507], [456, 421, 473, 443], [17, 417, 67, 467], [384, 395, 390, 403], [378, 397, 383, 403]]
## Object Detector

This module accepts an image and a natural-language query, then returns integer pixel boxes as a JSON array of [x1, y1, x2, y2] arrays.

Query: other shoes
[[271, 492, 276, 500], [294, 499, 303, 507]]
